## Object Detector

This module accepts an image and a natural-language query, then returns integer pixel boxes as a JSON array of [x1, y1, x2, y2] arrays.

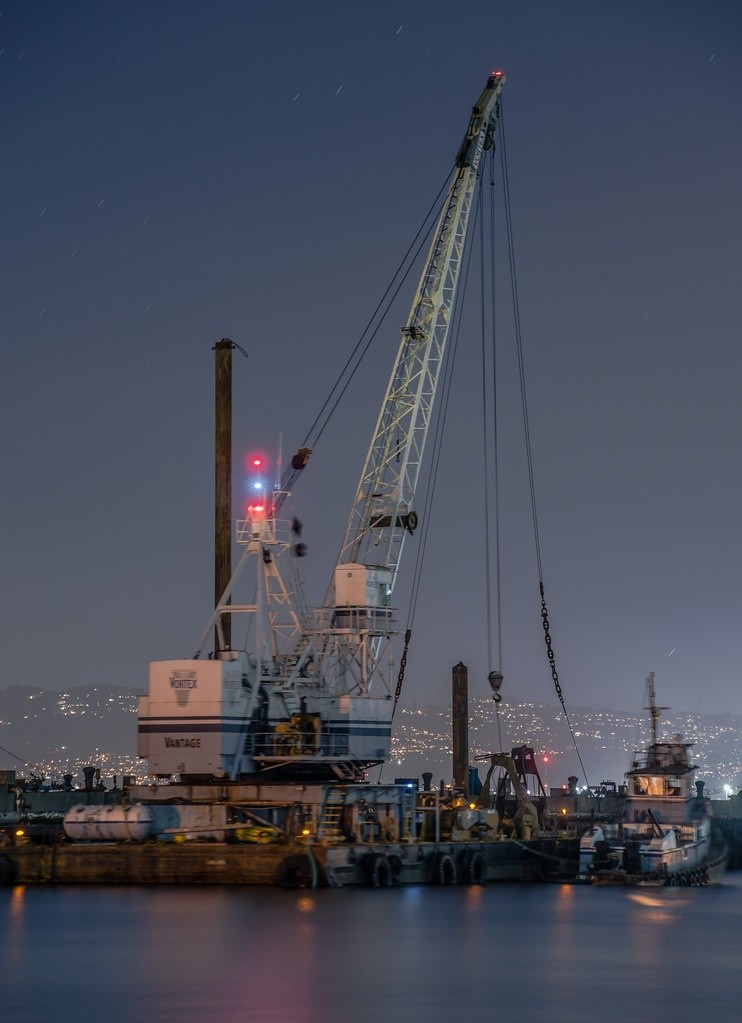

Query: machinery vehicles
[[130, 63, 574, 839]]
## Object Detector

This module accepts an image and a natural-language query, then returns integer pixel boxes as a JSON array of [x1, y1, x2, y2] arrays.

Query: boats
[[575, 670, 731, 892]]
